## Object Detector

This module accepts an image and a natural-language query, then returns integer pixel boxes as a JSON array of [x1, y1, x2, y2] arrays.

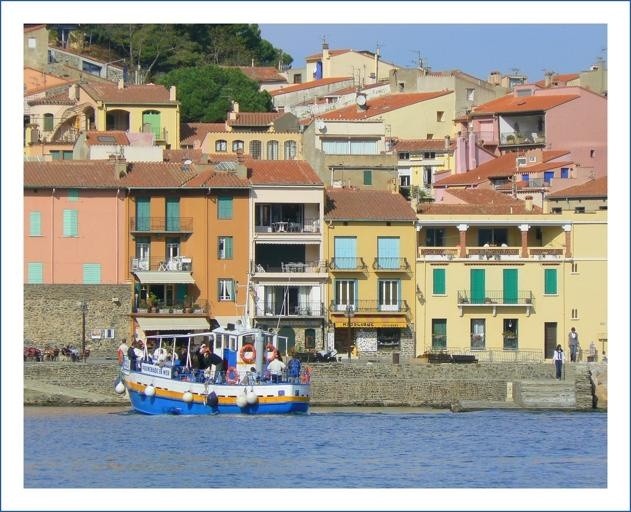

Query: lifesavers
[[263, 344, 278, 361], [240, 344, 255, 362], [118, 350, 123, 366], [226, 367, 238, 384]]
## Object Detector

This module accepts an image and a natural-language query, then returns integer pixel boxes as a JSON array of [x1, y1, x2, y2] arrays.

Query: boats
[[119, 323, 312, 416]]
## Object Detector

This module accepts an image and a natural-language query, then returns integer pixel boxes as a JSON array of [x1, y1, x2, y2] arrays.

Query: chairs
[[281, 261, 313, 273]]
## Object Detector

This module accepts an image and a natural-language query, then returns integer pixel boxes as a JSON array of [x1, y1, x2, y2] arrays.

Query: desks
[[272, 222, 290, 233]]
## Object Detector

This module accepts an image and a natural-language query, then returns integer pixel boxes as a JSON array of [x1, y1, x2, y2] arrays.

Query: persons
[[350, 343, 358, 359], [568, 326, 580, 361], [554, 344, 566, 380], [275, 351, 283, 362], [317, 347, 339, 358], [117, 338, 223, 369], [267, 354, 287, 383], [24, 345, 91, 362]]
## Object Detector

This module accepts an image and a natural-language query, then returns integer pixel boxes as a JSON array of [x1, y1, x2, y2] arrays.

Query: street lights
[[80, 303, 88, 364]]
